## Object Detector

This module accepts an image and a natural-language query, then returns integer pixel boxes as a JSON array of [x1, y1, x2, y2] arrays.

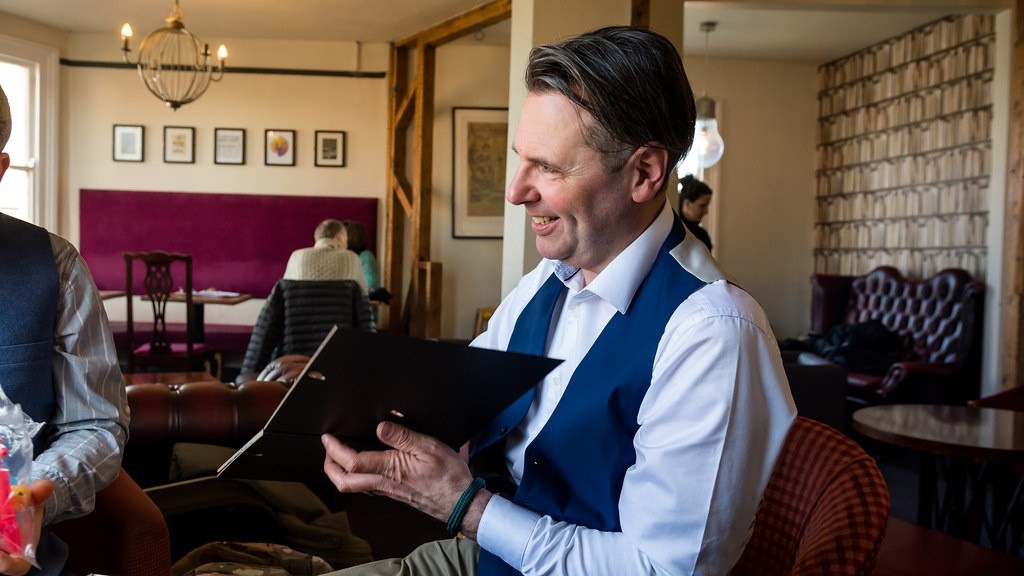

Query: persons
[[0, 88, 172, 576], [344, 222, 379, 322], [676, 174, 714, 253], [319, 26, 799, 576], [284, 219, 368, 294]]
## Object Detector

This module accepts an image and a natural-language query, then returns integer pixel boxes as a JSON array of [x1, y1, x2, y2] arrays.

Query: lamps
[[121, 0, 229, 113]]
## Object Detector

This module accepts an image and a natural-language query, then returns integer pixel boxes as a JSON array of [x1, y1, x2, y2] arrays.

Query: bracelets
[[446, 477, 486, 535]]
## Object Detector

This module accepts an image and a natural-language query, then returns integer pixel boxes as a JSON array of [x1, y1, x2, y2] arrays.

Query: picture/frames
[[264, 129, 296, 166], [314, 129, 346, 167], [112, 124, 144, 162], [163, 125, 195, 164], [450, 107, 509, 239], [214, 128, 244, 165]]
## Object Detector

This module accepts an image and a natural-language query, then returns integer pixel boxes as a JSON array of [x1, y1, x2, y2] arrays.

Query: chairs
[[53, 464, 172, 576], [122, 249, 220, 383], [241, 278, 378, 375], [724, 414, 889, 576]]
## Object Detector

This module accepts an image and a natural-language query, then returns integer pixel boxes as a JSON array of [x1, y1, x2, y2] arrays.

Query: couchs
[[813, 268, 985, 408], [125, 354, 335, 471]]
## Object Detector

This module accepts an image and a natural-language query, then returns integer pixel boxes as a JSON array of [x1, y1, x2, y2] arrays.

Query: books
[[142, 324, 562, 511]]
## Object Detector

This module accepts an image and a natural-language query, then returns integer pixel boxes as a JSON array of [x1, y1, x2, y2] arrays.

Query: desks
[[854, 403, 1024, 538], [141, 290, 253, 373]]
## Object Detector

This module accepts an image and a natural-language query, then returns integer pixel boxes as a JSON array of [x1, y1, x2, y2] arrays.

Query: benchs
[[78, 187, 379, 353]]
